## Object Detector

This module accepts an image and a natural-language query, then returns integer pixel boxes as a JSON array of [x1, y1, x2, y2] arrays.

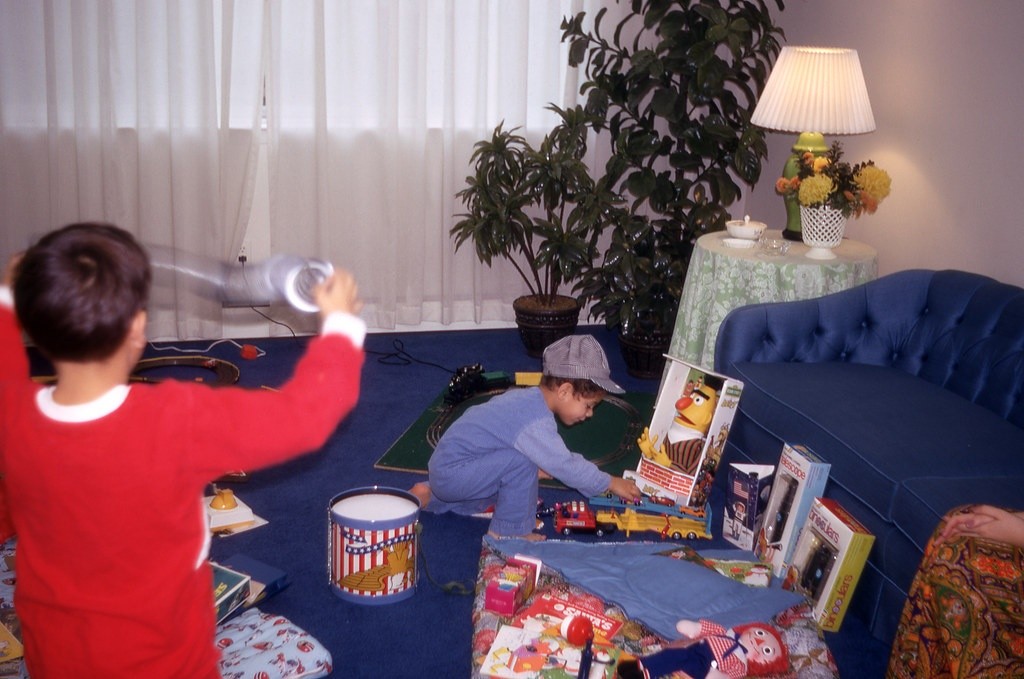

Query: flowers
[[775, 139, 892, 219]]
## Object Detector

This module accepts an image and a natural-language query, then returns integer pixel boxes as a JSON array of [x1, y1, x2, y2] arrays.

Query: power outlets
[[234, 242, 250, 269]]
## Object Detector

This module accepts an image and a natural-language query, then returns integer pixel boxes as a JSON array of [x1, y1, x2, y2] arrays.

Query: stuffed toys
[[617, 613, 791, 679]]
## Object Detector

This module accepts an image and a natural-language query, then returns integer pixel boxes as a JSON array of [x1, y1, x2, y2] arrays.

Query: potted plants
[[447, 0, 793, 379]]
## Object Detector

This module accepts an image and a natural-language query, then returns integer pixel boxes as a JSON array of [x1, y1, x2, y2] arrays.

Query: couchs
[[708, 266, 1024, 660]]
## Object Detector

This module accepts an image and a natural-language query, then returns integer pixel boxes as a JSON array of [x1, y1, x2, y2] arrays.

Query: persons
[[426, 330, 640, 558], [0, 224, 367, 679]]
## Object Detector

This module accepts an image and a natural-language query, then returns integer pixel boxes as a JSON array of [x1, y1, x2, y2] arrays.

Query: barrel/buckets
[[324, 485, 422, 605]]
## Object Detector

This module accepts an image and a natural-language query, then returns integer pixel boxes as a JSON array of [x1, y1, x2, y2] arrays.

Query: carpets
[[374, 374, 653, 493], [470, 534, 839, 679]]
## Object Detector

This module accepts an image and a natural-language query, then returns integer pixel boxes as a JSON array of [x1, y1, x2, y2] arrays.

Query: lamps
[[750, 44, 879, 239]]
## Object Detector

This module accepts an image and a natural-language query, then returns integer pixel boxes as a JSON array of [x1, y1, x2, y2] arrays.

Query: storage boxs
[[483, 552, 544, 617], [629, 354, 876, 636], [210, 563, 250, 629]]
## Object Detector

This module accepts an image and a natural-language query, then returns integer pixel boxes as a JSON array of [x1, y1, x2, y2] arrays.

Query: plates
[[723, 239, 755, 248]]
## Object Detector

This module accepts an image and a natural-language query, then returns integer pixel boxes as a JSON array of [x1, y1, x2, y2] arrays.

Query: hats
[[543, 334, 626, 394]]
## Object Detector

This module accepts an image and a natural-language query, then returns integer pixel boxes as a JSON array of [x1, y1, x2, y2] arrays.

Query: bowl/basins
[[725, 220, 767, 241], [757, 238, 791, 257]]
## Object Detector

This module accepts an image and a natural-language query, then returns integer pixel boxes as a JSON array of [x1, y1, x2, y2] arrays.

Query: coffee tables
[[658, 230, 879, 377]]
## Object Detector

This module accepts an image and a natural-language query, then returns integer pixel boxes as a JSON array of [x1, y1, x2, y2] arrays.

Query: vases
[[797, 198, 847, 261]]
